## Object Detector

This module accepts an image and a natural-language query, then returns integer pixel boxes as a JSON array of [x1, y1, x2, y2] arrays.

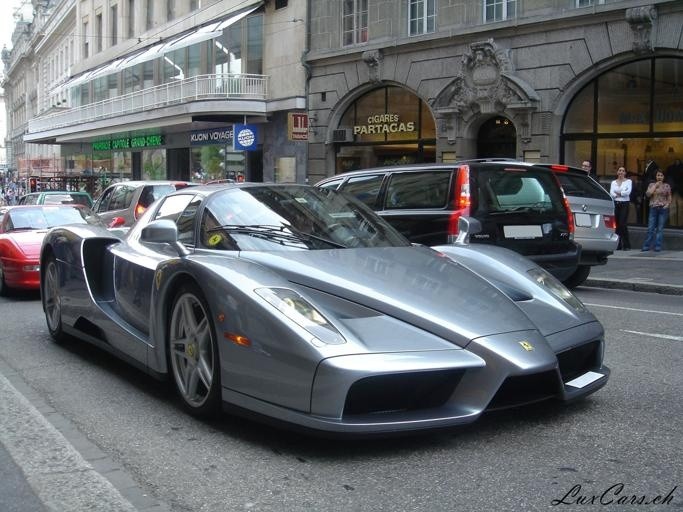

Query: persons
[[610, 166, 632, 251], [641, 168, 672, 251], [642, 159, 683, 201], [0, 177, 16, 205], [581, 159, 600, 183]]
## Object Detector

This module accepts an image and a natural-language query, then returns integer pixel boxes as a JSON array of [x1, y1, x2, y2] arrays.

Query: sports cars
[[39, 182, 610, 434]]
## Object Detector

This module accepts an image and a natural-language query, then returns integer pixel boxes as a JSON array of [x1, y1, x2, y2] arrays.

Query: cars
[[0, 182, 203, 294]]
